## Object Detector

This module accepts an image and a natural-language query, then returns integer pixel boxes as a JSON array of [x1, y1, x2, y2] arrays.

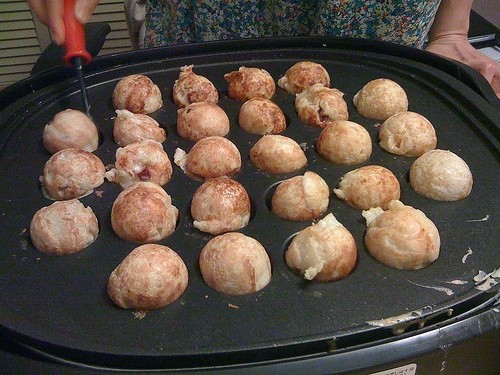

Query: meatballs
[[29, 61, 472, 309]]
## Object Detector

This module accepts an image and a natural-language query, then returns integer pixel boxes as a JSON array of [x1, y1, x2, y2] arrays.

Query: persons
[[30, 0, 500, 99]]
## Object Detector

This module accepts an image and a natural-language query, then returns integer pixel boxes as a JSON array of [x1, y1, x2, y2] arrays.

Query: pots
[[0, 21, 500, 374]]
